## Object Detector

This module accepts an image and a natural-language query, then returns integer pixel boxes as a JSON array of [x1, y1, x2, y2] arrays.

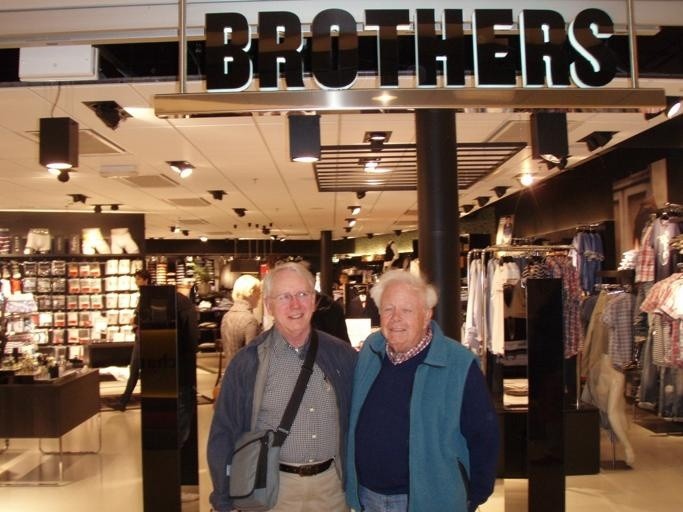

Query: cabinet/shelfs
[[0, 254, 214, 360]]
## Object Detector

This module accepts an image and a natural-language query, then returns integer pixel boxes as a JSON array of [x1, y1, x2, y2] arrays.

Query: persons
[[80, 226, 109, 255], [205, 261, 359, 512], [340, 269, 500, 512], [22, 228, 53, 254], [109, 227, 139, 255], [219, 272, 263, 367], [104, 269, 158, 412], [310, 288, 352, 350], [381, 238, 399, 265]]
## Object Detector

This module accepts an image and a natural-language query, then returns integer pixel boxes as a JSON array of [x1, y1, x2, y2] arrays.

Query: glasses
[[267, 290, 312, 302]]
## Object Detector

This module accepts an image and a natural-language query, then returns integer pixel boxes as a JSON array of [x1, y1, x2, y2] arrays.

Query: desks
[[0, 369, 103, 486], [504, 378, 600, 478]]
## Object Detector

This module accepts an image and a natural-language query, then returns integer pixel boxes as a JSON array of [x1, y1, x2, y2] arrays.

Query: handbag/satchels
[[227, 428, 281, 512]]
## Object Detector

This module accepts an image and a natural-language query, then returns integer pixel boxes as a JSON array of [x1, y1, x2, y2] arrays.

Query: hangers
[[595, 283, 632, 294], [471, 245, 570, 264], [575, 224, 598, 235]]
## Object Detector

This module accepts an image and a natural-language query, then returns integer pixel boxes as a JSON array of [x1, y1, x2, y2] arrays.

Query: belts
[[277, 458, 334, 477]]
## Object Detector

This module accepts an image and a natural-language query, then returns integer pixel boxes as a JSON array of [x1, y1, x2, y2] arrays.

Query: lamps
[[287, 114, 321, 162], [342, 131, 392, 240], [460, 99, 680, 217], [39, 86, 271, 240]]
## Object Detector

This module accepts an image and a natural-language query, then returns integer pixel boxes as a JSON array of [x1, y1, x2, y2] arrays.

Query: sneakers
[[103, 397, 126, 412]]
